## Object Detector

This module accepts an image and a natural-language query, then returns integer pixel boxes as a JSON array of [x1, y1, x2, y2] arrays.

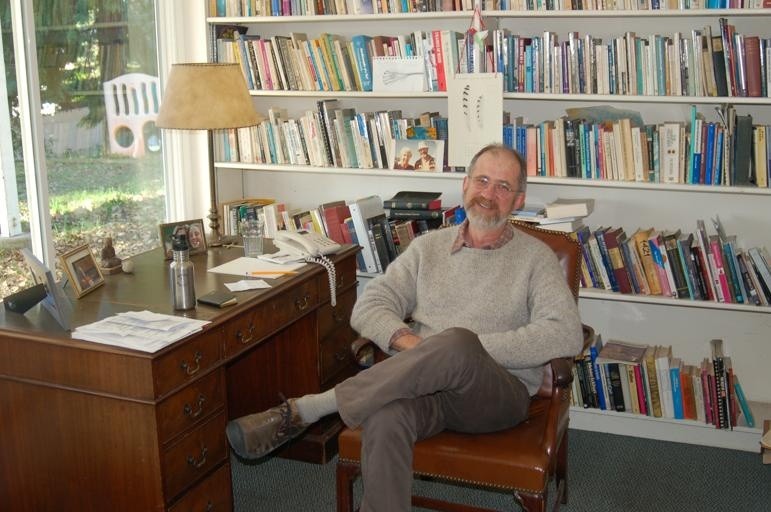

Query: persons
[[395, 148, 414, 170], [414, 142, 435, 171], [227, 145, 584, 512]]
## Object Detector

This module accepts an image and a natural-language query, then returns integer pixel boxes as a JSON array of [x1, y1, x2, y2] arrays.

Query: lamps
[[155, 65, 260, 248]]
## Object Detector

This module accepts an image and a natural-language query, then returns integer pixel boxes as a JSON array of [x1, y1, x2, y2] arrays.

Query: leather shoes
[[225, 397, 311, 460]]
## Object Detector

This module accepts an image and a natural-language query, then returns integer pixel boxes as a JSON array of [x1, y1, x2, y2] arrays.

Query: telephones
[[272, 229, 343, 260]]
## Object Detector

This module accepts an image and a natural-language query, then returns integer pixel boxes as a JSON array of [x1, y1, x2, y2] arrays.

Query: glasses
[[468, 175, 525, 193]]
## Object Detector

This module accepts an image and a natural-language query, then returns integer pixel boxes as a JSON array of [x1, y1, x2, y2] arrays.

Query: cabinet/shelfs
[[204, 0, 771, 432]]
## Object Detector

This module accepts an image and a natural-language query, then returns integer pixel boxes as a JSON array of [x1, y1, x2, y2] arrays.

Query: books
[[215, 24, 471, 91], [209, 2, 767, 16], [568, 321, 767, 434], [218, 100, 449, 170], [482, 23, 767, 97], [498, 105, 767, 186], [502, 196, 767, 305], [218, 192, 461, 274]]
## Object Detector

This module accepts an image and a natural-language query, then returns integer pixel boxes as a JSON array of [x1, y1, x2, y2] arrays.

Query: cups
[[241, 221, 265, 258]]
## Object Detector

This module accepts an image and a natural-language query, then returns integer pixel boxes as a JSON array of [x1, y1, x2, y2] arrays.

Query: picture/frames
[[62, 243, 105, 297], [3, 250, 71, 334], [160, 219, 208, 261]]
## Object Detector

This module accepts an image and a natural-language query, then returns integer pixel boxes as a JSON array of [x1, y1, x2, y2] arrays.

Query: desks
[[0, 231, 364, 512]]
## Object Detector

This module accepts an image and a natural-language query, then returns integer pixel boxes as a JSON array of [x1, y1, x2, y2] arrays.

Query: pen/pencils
[[245, 272, 301, 276]]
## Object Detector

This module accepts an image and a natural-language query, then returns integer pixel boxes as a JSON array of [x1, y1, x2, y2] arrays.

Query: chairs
[[338, 217, 581, 512]]
